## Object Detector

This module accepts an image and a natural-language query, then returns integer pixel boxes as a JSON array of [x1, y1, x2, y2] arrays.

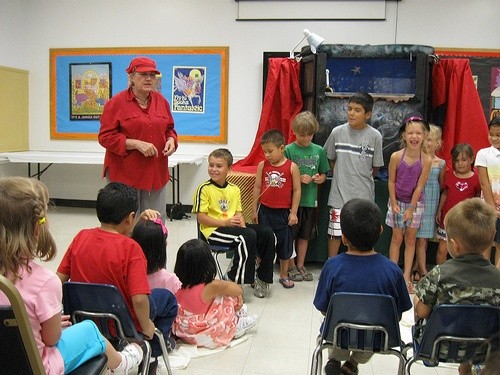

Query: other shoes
[[407, 282, 415, 294]]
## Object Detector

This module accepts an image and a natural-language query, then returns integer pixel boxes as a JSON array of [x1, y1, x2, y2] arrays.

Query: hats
[[126, 57, 160, 75], [398, 112, 430, 138]]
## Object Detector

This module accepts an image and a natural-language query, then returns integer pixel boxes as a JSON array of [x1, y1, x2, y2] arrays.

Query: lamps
[[290, 29, 325, 60]]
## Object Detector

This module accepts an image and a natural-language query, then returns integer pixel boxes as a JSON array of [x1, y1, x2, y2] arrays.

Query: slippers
[[410, 271, 420, 282], [279, 277, 295, 288]]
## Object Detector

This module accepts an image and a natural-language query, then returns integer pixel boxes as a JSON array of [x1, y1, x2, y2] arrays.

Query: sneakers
[[235, 304, 247, 317], [298, 266, 313, 281], [160, 337, 176, 355], [288, 269, 303, 281], [233, 315, 258, 338], [254, 273, 267, 298]]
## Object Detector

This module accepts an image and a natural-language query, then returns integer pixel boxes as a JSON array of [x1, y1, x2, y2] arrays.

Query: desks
[[0, 151, 203, 221]]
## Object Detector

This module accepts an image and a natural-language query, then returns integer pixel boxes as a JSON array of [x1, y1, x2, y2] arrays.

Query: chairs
[[197, 219, 236, 280], [311, 292, 407, 375], [407, 304, 500, 375], [0, 274, 109, 375], [62, 282, 171, 375]]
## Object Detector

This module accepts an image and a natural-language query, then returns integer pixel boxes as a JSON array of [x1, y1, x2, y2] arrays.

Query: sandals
[[325, 359, 341, 375], [340, 360, 358, 375], [107, 342, 143, 375]]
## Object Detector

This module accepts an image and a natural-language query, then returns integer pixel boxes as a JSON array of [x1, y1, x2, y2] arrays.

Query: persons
[[173, 239, 259, 350], [410, 123, 446, 282], [312, 198, 411, 375], [387, 111, 432, 294], [191, 149, 274, 298], [253, 129, 301, 288], [411, 198, 500, 340], [131, 208, 181, 295], [475, 115, 500, 269], [0, 177, 143, 375], [283, 111, 331, 281], [98, 56, 178, 228], [55, 182, 177, 357], [323, 93, 385, 259], [436, 143, 481, 266]]
